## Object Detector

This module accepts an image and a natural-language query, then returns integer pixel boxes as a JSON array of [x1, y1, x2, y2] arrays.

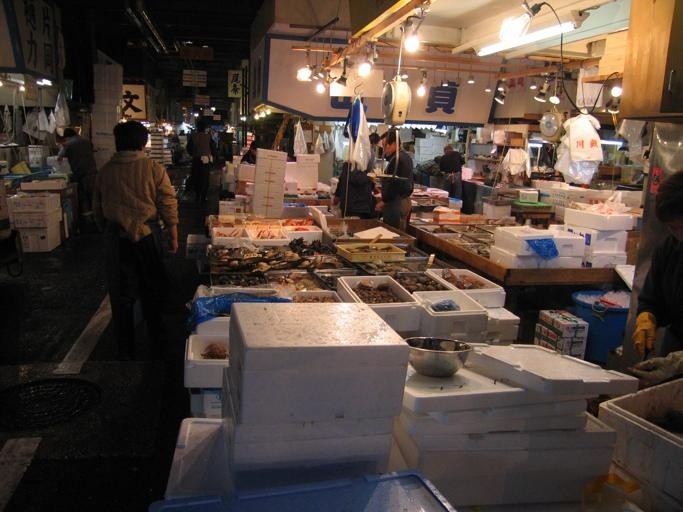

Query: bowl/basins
[[403, 336, 471, 378]]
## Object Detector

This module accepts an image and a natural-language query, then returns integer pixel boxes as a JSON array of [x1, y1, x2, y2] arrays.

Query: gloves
[[632, 312, 657, 358]]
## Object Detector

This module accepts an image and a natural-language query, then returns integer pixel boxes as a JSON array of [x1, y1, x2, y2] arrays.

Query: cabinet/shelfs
[[617, 0, 683, 123]]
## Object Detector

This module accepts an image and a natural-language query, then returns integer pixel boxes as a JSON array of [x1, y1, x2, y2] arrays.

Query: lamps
[[297, 0, 622, 105]]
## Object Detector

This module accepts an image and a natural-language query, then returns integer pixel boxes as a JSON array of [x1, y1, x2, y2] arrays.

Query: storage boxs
[[149, 137, 683, 512], [0, 142, 80, 254]]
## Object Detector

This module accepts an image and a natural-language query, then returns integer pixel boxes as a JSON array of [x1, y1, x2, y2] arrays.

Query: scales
[[366, 75, 411, 199], [530, 111, 564, 180]]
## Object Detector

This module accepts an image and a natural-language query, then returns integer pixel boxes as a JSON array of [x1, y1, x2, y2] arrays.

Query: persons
[[91, 119, 179, 362], [628, 169, 683, 387], [332, 132, 466, 233], [168, 115, 258, 208], [56, 128, 98, 217]]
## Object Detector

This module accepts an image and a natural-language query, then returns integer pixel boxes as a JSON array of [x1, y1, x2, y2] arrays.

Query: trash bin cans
[[572, 290, 631, 362]]
